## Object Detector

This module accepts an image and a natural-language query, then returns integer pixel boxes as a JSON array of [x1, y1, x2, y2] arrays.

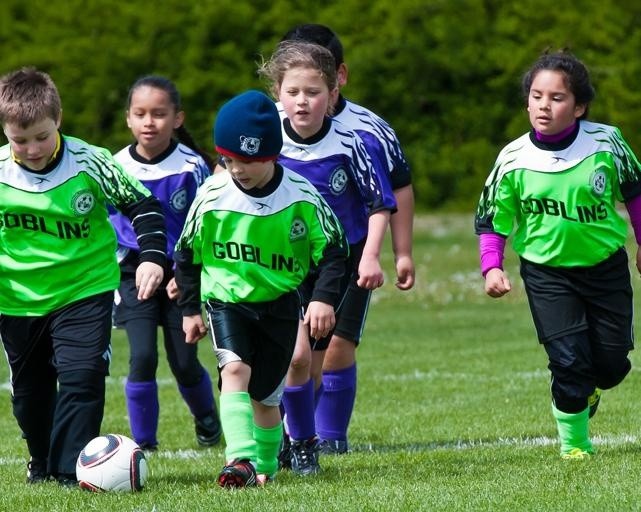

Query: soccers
[[76, 434, 147, 493]]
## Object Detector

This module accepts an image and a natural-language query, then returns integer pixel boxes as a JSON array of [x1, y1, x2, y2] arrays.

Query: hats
[[214, 90, 283, 164]]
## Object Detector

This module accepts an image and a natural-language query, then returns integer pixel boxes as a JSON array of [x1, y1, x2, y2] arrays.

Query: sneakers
[[25, 455, 47, 484], [217, 457, 256, 490], [321, 438, 346, 454], [589, 387, 601, 419], [194, 397, 222, 447], [277, 413, 324, 474]]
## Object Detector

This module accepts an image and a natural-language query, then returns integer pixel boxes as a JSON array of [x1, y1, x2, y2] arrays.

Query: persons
[[474, 53, 640, 462], [256, 41, 398, 483], [106, 74, 221, 456], [172, 89, 352, 489], [272, 23, 415, 458], [0, 64, 167, 489]]
